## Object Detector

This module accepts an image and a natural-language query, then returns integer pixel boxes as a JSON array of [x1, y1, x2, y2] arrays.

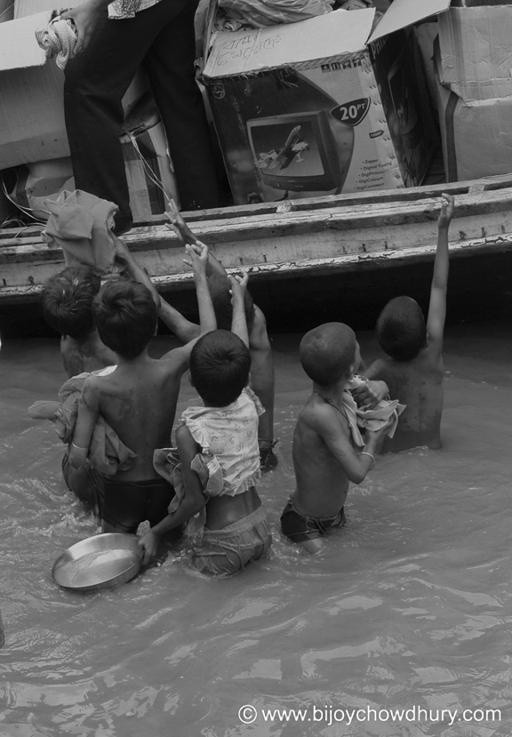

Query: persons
[[43, 248, 117, 380], [56, 0, 234, 237], [353, 190, 456, 456], [62, 240, 221, 550], [136, 267, 274, 578], [278, 319, 394, 556], [106, 197, 279, 477]]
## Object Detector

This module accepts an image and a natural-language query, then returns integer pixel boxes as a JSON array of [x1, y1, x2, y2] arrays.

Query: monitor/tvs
[[245, 109, 340, 192]]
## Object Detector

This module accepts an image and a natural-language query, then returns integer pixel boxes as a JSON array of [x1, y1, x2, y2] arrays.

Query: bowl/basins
[[52, 533, 144, 592]]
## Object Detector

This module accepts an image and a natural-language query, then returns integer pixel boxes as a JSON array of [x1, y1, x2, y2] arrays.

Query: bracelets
[[361, 451, 376, 463]]
